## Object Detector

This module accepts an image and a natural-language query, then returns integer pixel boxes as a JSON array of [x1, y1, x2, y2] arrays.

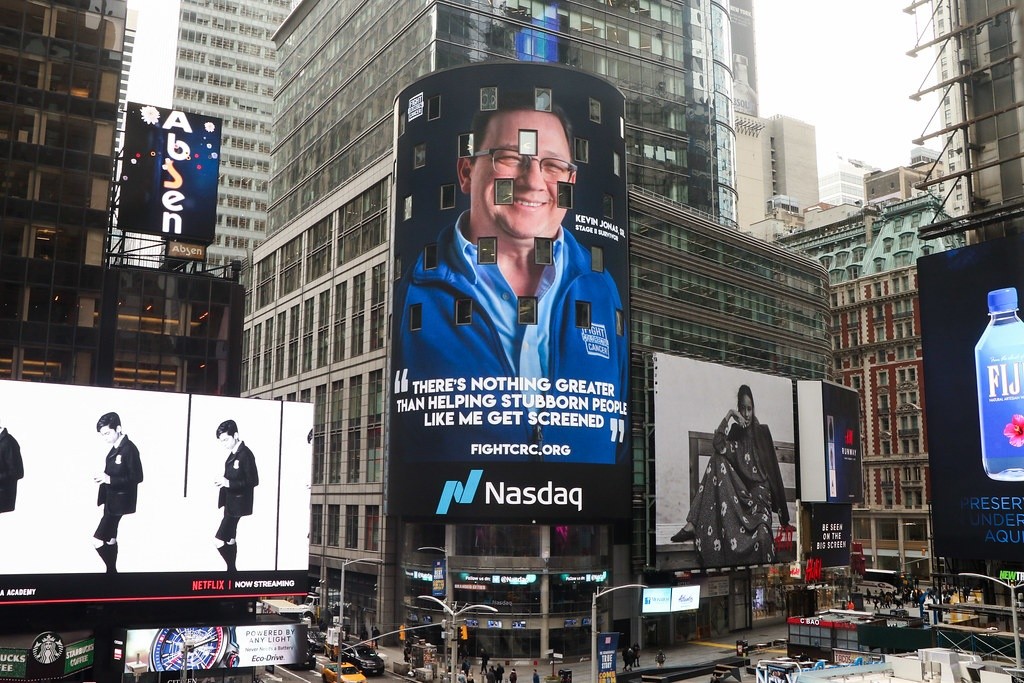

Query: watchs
[[148, 626, 240, 672]]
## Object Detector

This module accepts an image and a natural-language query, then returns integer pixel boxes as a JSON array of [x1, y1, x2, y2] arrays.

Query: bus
[[261, 599, 304, 620], [851, 569, 900, 595]]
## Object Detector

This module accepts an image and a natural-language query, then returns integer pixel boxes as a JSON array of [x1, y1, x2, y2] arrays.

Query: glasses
[[469, 148, 577, 184]]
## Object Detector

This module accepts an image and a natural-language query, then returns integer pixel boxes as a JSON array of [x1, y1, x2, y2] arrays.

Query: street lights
[[417, 596, 498, 683], [337, 557, 384, 683], [591, 584, 648, 683], [418, 546, 452, 673], [959, 573, 1022, 683]]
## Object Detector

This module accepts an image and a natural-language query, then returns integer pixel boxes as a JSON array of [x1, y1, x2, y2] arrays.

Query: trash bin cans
[[342, 625, 351, 641], [424, 662, 438, 678], [737, 640, 748, 656], [558, 669, 572, 683]]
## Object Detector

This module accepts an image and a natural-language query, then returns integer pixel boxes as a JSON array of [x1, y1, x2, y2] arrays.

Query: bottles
[[975, 288, 1024, 482]]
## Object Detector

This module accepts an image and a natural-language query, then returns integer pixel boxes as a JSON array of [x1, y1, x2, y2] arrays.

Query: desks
[[772, 655, 850, 670]]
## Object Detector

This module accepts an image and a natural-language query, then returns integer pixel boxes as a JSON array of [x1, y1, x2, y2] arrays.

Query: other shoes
[[671, 529, 695, 543]]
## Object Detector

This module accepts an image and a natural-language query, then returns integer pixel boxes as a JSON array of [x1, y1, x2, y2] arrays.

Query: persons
[[915, 576, 919, 588], [215, 419, 259, 570], [390, 90, 626, 465], [479, 653, 489, 674], [866, 585, 971, 611], [373, 627, 380, 649], [671, 384, 796, 565], [360, 630, 368, 641], [622, 641, 640, 672], [532, 669, 539, 683], [486, 663, 504, 683], [655, 650, 666, 666], [509, 669, 517, 683], [93, 413, 143, 572], [458, 659, 474, 683], [842, 601, 854, 610]]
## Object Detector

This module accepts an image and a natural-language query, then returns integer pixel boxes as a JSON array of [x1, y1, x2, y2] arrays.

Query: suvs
[[299, 592, 319, 626]]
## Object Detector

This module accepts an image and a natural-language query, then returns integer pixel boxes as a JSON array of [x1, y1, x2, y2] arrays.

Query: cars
[[341, 642, 385, 678]]
